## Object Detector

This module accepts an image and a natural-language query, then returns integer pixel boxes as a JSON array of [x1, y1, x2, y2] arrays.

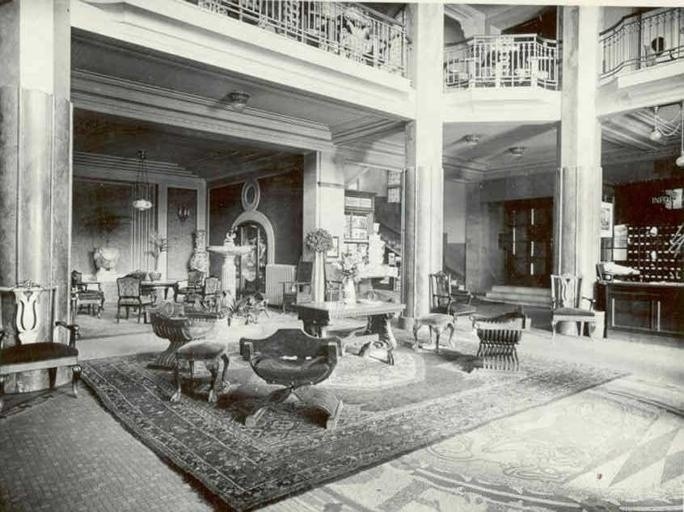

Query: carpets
[[71, 321, 633, 512]]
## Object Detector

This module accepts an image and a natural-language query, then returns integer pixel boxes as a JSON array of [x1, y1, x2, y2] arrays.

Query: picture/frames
[[325, 234, 339, 258]]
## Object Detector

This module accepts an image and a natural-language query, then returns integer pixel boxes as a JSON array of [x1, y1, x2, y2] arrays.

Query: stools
[[411, 314, 458, 353], [170, 341, 231, 404]]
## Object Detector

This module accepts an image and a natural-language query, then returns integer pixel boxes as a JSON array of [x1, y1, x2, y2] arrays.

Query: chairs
[[2, 282, 80, 398], [432, 271, 478, 343], [550, 274, 599, 338], [69, 261, 400, 334], [239, 328, 344, 431], [469, 305, 531, 371], [148, 300, 218, 370], [215, 1, 404, 68], [446, 41, 562, 95]]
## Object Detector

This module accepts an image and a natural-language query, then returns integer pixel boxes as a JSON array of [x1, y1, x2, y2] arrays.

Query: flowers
[[303, 226, 334, 254]]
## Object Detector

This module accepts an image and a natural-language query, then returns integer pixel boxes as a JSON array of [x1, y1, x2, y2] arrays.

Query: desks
[[292, 300, 406, 366]]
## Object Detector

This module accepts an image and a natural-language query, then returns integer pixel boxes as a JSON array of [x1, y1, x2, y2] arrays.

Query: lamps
[[675, 99, 684, 167], [647, 104, 663, 142], [128, 149, 153, 212], [226, 90, 251, 115]]
